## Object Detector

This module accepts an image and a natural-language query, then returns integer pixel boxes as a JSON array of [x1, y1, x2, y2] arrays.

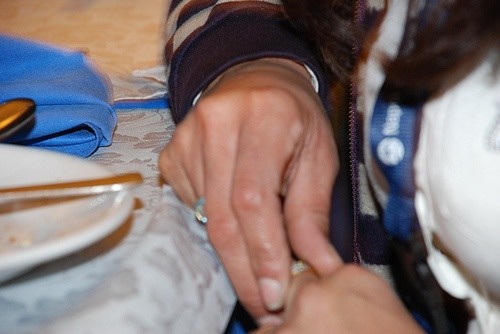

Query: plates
[[0, 143, 134, 284]]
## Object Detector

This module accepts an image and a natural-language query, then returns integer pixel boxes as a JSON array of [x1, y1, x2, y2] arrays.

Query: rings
[[289, 259, 314, 281], [195, 197, 205, 223]]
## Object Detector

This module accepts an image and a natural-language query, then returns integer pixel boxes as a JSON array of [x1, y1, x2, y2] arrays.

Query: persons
[[160, 0, 500, 334]]
